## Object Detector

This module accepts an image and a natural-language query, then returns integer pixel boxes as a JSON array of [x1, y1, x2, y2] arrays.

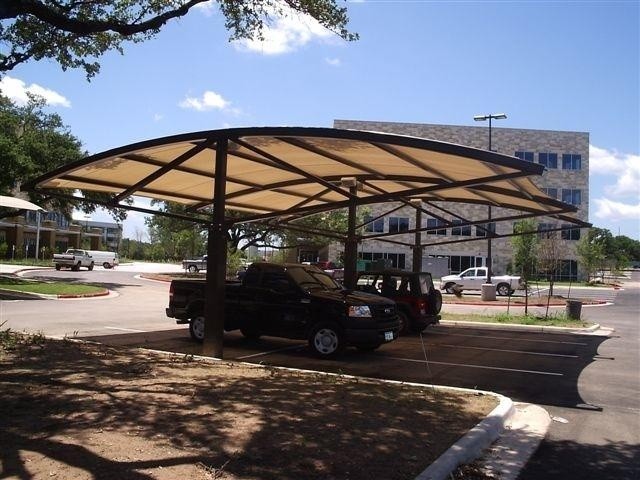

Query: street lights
[[474, 114, 507, 285]]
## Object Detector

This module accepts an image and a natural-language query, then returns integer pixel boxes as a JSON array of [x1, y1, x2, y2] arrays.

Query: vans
[[85, 250, 119, 268]]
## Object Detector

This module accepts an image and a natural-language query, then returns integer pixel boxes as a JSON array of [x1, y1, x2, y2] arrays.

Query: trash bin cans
[[566, 300, 582, 320]]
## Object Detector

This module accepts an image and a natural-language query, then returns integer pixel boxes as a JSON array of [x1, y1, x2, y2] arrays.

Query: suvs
[[333, 268, 443, 335]]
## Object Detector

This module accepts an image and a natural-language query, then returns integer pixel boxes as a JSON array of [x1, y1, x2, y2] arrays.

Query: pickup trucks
[[440, 266, 523, 296], [182, 255, 209, 273], [52, 249, 95, 271], [164, 262, 404, 359]]
